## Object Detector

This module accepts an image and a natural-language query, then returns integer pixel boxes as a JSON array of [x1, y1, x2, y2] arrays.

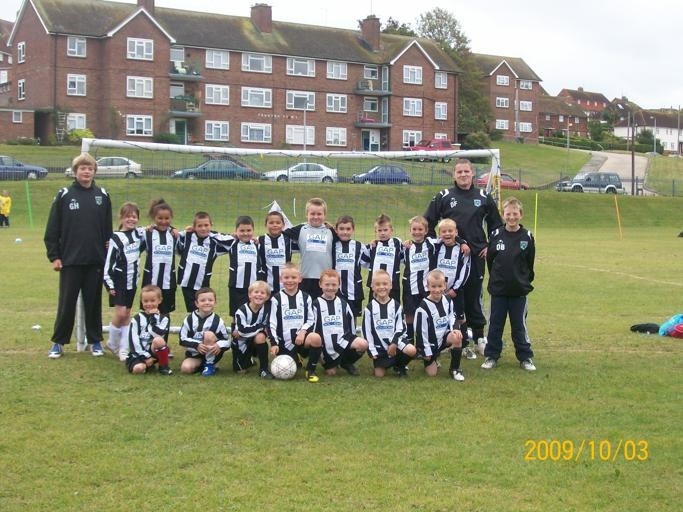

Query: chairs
[[297, 352, 304, 368], [449, 368, 465, 381], [341, 362, 360, 375], [462, 347, 476, 359], [106, 340, 129, 360], [260, 369, 275, 379], [521, 359, 536, 371], [49, 343, 64, 358], [478, 342, 487, 355], [394, 363, 408, 377], [305, 371, 319, 382], [161, 366, 173, 375], [202, 363, 215, 376], [481, 357, 498, 369], [90, 341, 104, 356]]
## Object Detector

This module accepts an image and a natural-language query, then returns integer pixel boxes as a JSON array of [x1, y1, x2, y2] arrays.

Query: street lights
[[660, 314, 683, 337], [631, 323, 659, 333]]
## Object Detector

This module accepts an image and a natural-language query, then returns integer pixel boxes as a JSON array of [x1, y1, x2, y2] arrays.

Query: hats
[[472, 171, 528, 190], [0, 155, 48, 182], [64, 157, 142, 179], [171, 158, 256, 182], [261, 160, 340, 183], [346, 165, 412, 185]]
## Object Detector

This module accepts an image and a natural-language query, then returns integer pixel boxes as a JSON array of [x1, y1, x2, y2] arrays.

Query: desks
[[300, 95, 310, 159], [649, 114, 658, 152], [615, 103, 635, 194]]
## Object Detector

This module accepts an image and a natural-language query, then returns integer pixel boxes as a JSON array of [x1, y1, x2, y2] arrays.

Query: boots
[[402, 137, 455, 165], [556, 171, 622, 194]]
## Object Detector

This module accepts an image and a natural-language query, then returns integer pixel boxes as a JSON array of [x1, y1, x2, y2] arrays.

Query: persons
[[413, 269, 467, 380], [334, 212, 470, 355], [104, 200, 188, 361], [187, 200, 339, 323], [43, 153, 112, 359], [178, 287, 231, 376], [423, 160, 504, 354], [268, 263, 323, 379], [312, 268, 369, 376], [480, 197, 536, 371], [0, 190, 11, 227], [126, 285, 173, 375], [230, 281, 275, 378], [362, 270, 418, 379]]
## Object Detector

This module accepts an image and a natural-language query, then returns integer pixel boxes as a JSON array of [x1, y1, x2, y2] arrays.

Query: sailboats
[[14, 239, 22, 245], [271, 354, 297, 379]]
